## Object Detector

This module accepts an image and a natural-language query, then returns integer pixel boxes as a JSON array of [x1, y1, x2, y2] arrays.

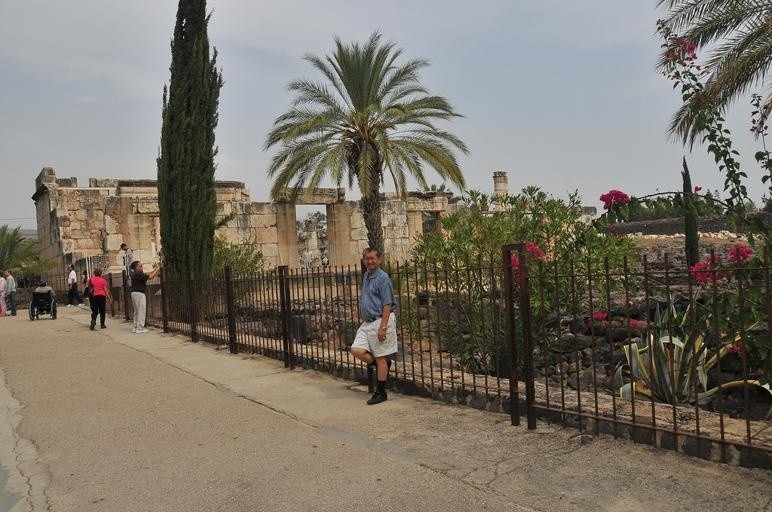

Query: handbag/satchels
[[84, 279, 94, 298]]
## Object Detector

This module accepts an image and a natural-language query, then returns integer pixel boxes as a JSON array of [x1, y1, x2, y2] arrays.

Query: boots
[[90, 318, 106, 330]]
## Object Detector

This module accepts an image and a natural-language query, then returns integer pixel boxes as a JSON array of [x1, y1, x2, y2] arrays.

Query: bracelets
[[380, 325, 388, 330]]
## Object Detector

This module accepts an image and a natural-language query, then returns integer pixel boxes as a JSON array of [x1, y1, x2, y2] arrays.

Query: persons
[[5, 268, 17, 316], [1, 270, 8, 316], [129, 261, 161, 334], [350, 247, 399, 405], [66, 264, 86, 307], [121, 243, 135, 288], [34, 278, 56, 314], [87, 268, 112, 330], [3, 272, 12, 315]]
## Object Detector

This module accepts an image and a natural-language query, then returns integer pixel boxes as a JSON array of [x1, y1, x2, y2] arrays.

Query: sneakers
[[132, 324, 149, 334], [367, 392, 388, 405], [66, 303, 86, 307]]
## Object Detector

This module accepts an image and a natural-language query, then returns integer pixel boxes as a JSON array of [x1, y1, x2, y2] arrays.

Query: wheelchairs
[[29, 290, 57, 320]]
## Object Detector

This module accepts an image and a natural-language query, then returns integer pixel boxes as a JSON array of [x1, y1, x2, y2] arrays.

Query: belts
[[366, 311, 393, 323]]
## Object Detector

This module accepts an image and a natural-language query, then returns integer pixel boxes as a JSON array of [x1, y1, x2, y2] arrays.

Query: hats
[[38, 278, 47, 286]]
